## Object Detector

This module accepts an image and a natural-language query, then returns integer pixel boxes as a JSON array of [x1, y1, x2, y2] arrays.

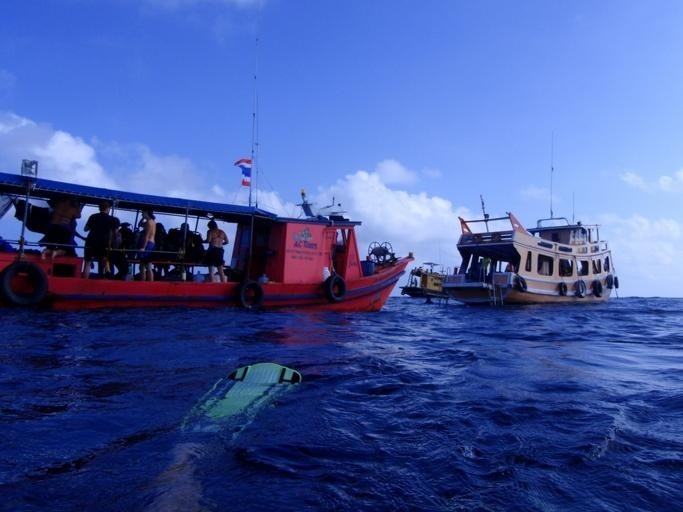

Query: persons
[[202, 220, 229, 284], [38, 196, 204, 281]]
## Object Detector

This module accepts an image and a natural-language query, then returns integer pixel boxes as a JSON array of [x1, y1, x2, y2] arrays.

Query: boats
[[400, 260, 457, 299], [0, 156, 415, 317], [441, 191, 621, 304]]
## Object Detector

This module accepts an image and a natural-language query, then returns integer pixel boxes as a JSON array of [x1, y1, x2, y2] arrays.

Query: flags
[[233, 157, 253, 189]]
